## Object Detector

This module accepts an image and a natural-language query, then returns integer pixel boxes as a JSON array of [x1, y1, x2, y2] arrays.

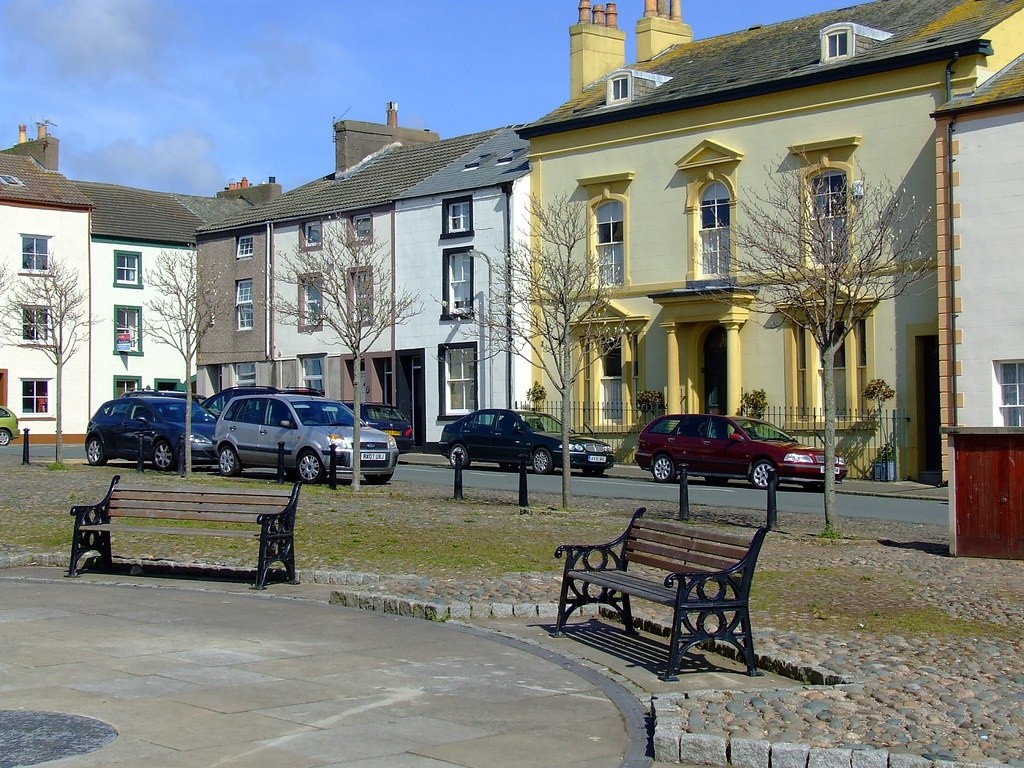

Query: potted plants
[[637, 389, 664, 424], [740, 387, 769, 426], [867, 442, 896, 481]]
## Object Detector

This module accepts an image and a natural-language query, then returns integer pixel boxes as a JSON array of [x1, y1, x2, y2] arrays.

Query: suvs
[[634, 413, 850, 491]]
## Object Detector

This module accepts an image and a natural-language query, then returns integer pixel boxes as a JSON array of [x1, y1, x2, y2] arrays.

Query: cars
[[82, 395, 218, 473], [114, 383, 414, 457], [213, 393, 398, 484], [0, 405, 21, 447], [438, 407, 615, 474]]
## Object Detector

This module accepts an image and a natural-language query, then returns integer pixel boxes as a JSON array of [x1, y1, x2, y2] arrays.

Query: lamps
[[851, 178, 865, 198]]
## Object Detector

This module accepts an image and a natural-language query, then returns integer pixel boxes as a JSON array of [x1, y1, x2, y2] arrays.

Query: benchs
[[550, 507, 772, 681], [62, 475, 303, 591]]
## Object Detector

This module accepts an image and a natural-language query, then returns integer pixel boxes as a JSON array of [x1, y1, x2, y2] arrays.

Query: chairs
[[678, 422, 705, 436]]
